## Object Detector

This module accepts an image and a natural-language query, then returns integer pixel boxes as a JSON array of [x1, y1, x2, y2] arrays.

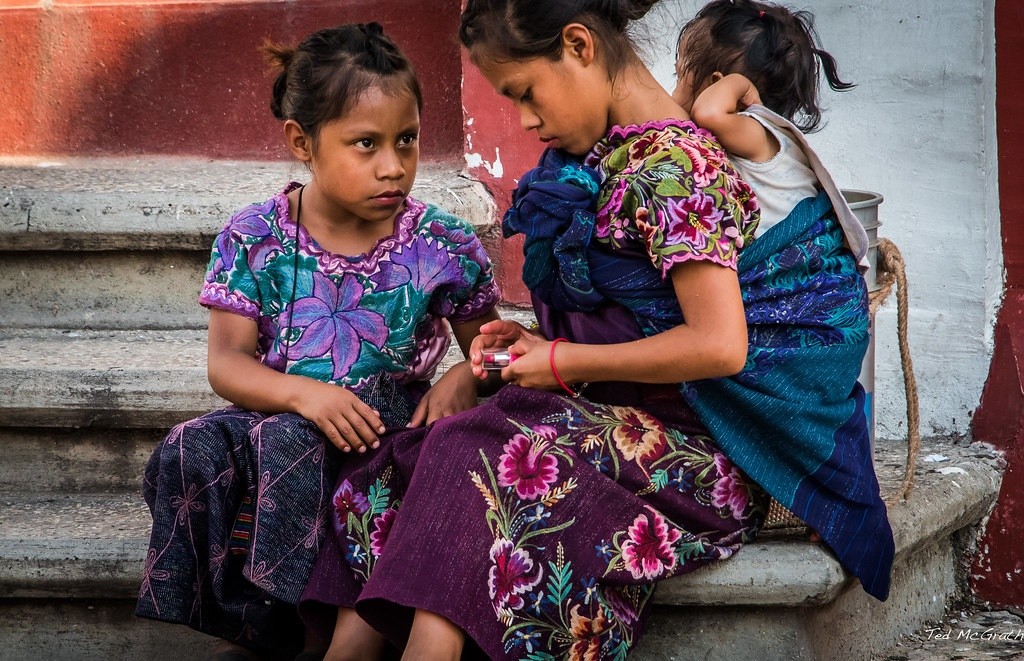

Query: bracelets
[[551, 337, 588, 400]]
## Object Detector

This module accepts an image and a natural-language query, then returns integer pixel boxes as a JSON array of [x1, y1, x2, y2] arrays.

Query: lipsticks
[[480, 348, 523, 370]]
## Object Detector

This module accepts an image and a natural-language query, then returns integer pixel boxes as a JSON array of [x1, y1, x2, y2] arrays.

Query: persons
[[135, 21, 509, 661], [671, 0, 870, 541], [297, 0, 761, 661]]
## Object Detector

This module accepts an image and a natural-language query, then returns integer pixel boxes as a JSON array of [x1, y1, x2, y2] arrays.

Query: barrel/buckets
[[839, 188, 883, 463]]
